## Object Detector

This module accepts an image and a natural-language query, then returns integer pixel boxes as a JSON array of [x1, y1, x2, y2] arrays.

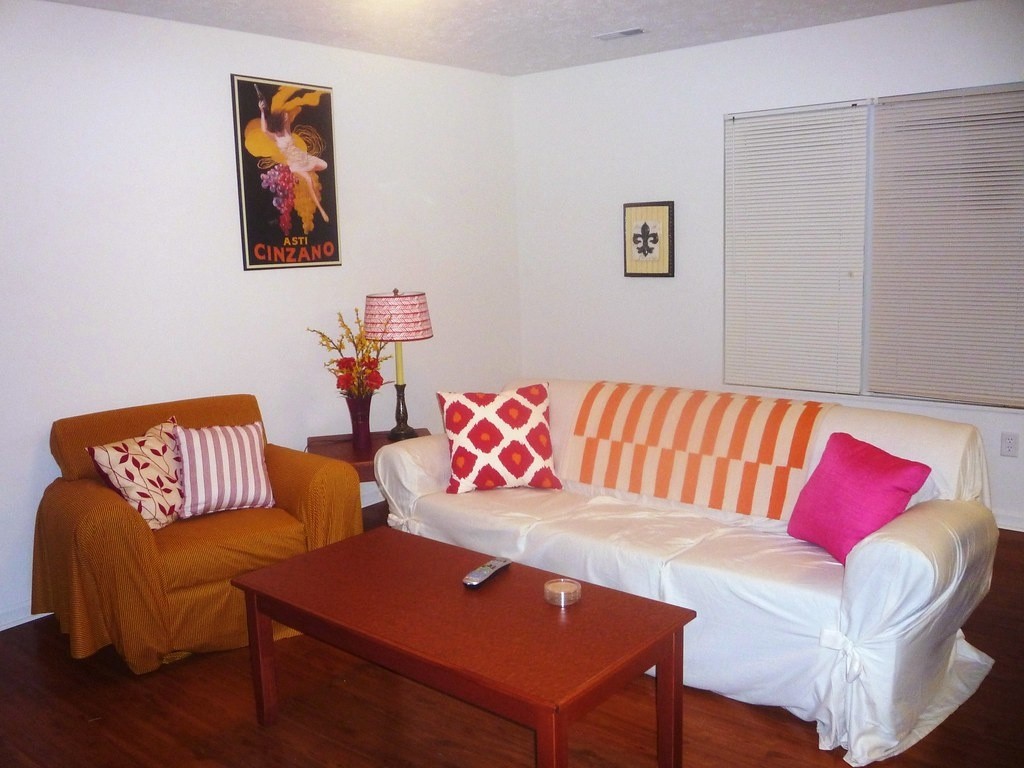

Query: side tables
[[306, 425, 433, 524]]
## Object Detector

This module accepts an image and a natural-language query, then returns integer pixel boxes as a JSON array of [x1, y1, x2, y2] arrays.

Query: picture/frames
[[624, 200, 674, 277], [229, 72, 343, 272]]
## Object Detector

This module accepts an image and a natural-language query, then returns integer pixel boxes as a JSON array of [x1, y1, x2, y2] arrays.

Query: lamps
[[362, 286, 434, 442]]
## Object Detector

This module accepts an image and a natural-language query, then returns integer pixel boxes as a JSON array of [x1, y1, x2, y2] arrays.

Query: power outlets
[[998, 433, 1020, 458]]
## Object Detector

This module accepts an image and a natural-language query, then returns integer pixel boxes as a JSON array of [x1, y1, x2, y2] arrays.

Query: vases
[[345, 392, 378, 454]]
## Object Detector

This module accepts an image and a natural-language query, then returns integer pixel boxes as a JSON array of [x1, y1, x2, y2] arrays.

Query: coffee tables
[[229, 522, 697, 768]]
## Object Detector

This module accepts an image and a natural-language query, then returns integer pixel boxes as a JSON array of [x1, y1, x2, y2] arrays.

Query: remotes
[[462, 557, 512, 588]]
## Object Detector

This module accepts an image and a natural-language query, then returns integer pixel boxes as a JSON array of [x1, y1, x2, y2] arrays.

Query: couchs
[[27, 391, 364, 675]]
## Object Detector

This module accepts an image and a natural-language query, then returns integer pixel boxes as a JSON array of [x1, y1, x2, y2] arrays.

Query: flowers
[[306, 307, 398, 398]]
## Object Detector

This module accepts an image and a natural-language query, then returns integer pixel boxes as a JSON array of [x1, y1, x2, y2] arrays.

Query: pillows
[[436, 379, 564, 498], [172, 414, 278, 521], [84, 414, 186, 530], [373, 374, 1001, 768], [786, 430, 934, 567]]
